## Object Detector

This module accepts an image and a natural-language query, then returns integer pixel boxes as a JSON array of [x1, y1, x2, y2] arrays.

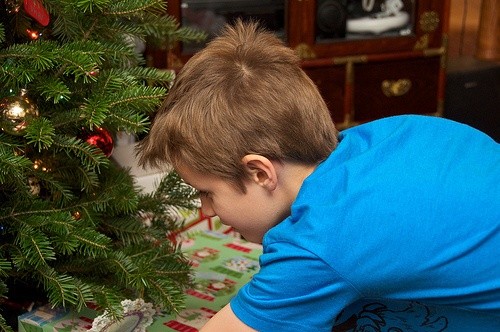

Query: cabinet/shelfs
[[148, 0, 452, 137]]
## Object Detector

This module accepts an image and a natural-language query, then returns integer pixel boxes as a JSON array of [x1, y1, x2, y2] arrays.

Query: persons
[[133, 17, 500, 332]]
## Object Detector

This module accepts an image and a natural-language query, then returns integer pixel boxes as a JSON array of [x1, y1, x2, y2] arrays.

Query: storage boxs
[[19, 230, 262, 332]]
